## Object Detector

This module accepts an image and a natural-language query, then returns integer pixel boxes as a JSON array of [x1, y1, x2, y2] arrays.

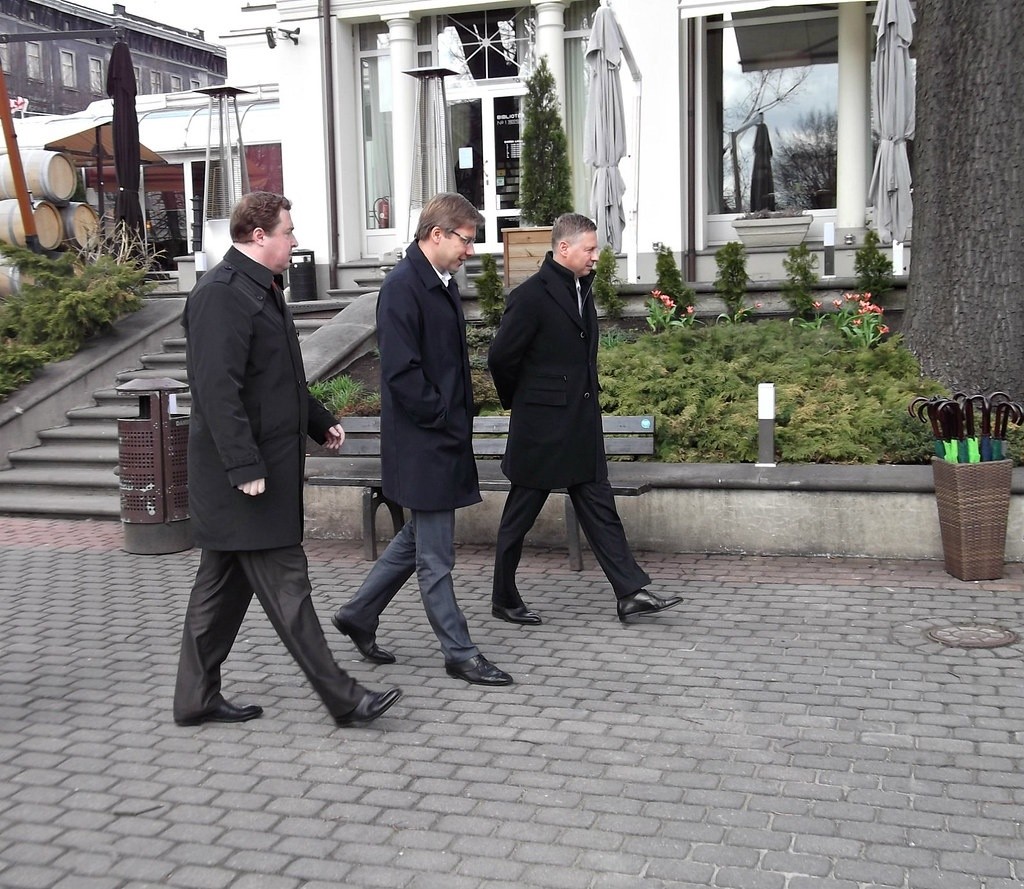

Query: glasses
[[430, 225, 476, 248]]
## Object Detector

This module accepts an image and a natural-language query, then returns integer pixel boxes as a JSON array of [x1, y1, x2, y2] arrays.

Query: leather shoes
[[331, 608, 396, 664], [175, 699, 264, 726], [492, 602, 542, 625], [617, 589, 684, 622], [334, 688, 402, 727], [445, 654, 513, 686]]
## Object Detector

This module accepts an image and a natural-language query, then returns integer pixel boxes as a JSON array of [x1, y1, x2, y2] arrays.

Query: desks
[[764, 189, 831, 195]]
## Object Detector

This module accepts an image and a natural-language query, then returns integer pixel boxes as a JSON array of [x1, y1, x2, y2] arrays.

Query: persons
[[173, 191, 402, 727], [489, 213, 684, 626], [330, 192, 513, 686]]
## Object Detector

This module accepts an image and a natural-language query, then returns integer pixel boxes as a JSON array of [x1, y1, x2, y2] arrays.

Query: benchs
[[310, 414, 656, 569]]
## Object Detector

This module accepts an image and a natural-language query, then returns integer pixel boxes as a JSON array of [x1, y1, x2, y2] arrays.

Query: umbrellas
[[106, 43, 146, 285], [750, 123, 775, 212], [908, 391, 1024, 464], [585, 5, 626, 255], [868, 0, 918, 244]]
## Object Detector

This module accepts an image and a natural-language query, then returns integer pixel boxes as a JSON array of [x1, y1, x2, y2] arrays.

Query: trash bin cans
[[116, 377, 196, 555], [288, 249, 317, 303]]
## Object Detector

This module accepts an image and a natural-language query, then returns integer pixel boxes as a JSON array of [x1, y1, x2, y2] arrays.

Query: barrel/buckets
[[0, 198, 63, 250], [0, 149, 77, 203], [0, 248, 87, 299], [57, 201, 101, 254]]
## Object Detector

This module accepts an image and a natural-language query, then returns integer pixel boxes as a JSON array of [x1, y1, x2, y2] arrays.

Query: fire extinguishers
[[373, 195, 389, 229]]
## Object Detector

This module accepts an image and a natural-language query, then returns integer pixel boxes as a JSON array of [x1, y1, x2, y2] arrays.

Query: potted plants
[[733, 204, 814, 248], [501, 54, 575, 289]]
